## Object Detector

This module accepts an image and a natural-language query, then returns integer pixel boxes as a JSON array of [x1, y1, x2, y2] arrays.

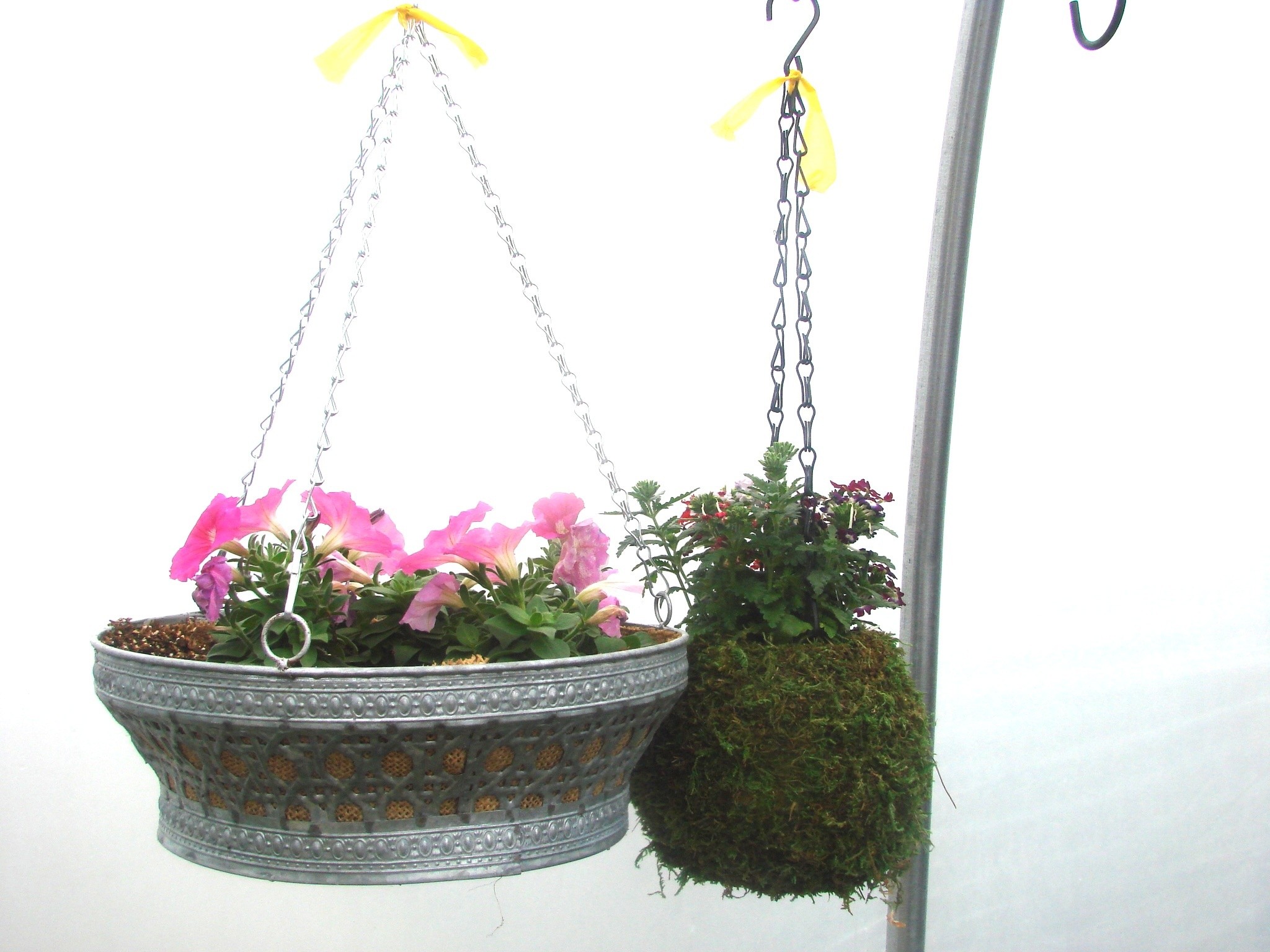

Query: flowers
[[609, 438, 908, 640], [161, 477, 651, 665]]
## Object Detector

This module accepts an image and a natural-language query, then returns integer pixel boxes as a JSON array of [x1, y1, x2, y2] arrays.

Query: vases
[[87, 609, 689, 886], [629, 635, 930, 915]]
